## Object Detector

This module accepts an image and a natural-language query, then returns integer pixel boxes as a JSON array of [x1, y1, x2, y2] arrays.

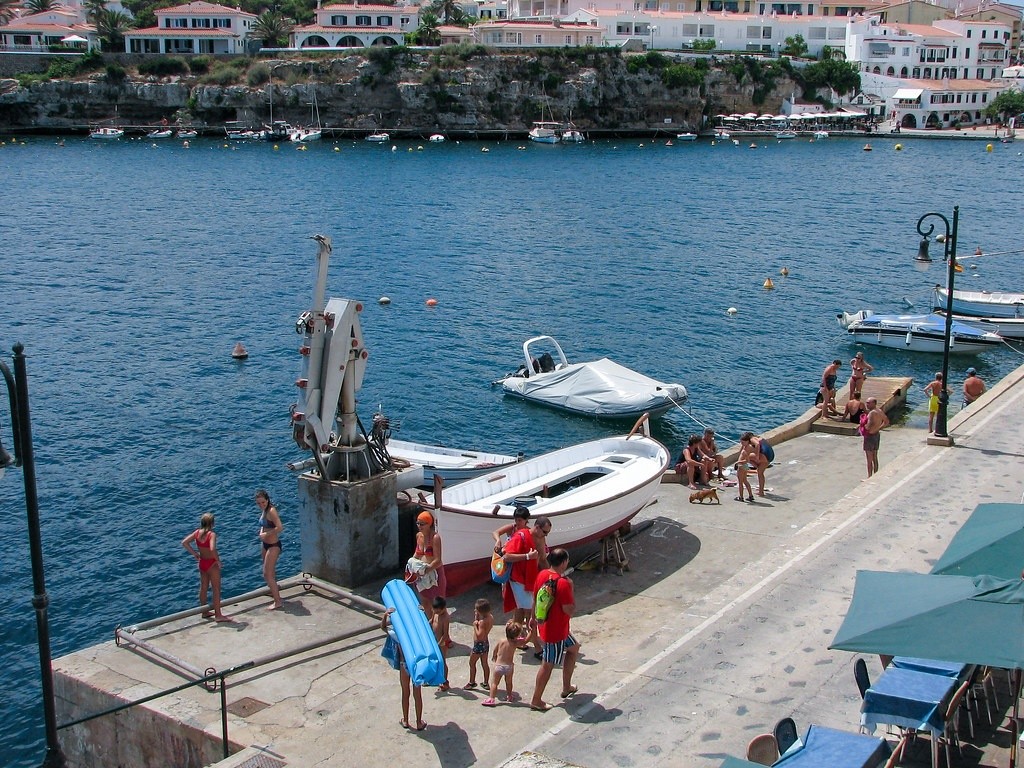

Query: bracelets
[[525, 553, 529, 560]]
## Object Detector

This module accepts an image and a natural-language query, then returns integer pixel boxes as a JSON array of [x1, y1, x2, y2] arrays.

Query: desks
[[858, 667, 960, 768], [892, 656, 982, 696], [770, 724, 893, 768]]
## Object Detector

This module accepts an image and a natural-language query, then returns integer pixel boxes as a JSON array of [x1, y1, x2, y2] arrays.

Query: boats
[[417, 413, 671, 596], [429, 135, 444, 142], [177, 131, 197, 138], [147, 130, 172, 138], [935, 310, 1024, 338], [528, 81, 563, 142], [358, 434, 523, 486], [562, 131, 582, 141], [836, 309, 1004, 355], [89, 127, 124, 139], [252, 130, 266, 139], [263, 64, 321, 141], [777, 129, 828, 139], [677, 132, 729, 140], [931, 284, 1024, 319], [492, 336, 688, 418], [368, 133, 390, 141], [224, 120, 253, 138]]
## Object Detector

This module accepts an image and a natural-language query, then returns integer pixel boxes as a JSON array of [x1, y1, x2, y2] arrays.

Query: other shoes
[[561, 684, 577, 698], [534, 580, 557, 624], [735, 497, 744, 502], [480, 682, 491, 690], [531, 702, 553, 711], [506, 694, 514, 702], [745, 496, 754, 501], [481, 699, 497, 706], [463, 683, 477, 689]]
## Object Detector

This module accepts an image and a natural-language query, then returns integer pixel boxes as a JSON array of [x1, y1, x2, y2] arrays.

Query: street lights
[[913, 212, 959, 437], [647, 25, 657, 49]]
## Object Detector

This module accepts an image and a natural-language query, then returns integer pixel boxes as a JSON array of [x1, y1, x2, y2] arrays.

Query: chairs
[[747, 734, 779, 766], [774, 717, 797, 756], [854, 654, 1013, 768]]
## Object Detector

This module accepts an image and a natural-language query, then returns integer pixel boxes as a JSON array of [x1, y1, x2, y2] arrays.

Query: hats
[[965, 367, 977, 374]]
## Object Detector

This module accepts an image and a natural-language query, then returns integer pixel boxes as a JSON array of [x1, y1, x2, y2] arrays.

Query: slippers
[[400, 718, 409, 729], [447, 641, 454, 648], [417, 720, 427, 731]]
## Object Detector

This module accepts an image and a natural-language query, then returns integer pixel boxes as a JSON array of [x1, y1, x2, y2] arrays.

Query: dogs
[[689, 488, 720, 504]]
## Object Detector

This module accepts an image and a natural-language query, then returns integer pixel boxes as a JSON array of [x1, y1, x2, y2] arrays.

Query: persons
[[836, 391, 866, 424], [183, 513, 234, 622], [962, 368, 988, 407], [861, 397, 890, 482], [698, 427, 728, 480], [850, 352, 873, 400], [381, 605, 427, 730], [463, 599, 493, 690], [924, 372, 954, 433], [482, 621, 532, 707], [815, 359, 846, 422], [529, 549, 579, 711], [404, 510, 450, 692], [733, 430, 774, 502], [492, 507, 551, 661], [254, 489, 283, 611], [674, 433, 716, 491], [161, 116, 168, 131]]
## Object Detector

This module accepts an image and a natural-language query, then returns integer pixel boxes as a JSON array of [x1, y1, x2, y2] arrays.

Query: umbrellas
[[713, 110, 868, 131], [828, 568, 1023, 768], [61, 34, 88, 48], [930, 501, 1024, 580]]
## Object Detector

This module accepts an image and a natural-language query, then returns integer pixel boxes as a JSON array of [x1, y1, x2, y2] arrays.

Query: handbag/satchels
[[491, 550, 512, 585]]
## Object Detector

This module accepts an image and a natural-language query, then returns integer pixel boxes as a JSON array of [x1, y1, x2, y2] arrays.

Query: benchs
[[462, 452, 657, 515]]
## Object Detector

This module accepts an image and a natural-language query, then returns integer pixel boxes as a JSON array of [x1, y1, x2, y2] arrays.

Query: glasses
[[540, 528, 550, 534], [416, 523, 428, 526], [854, 357, 861, 359]]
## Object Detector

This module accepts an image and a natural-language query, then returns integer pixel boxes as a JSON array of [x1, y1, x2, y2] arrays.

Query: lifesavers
[[390, 456, 410, 468], [382, 579, 446, 687]]
